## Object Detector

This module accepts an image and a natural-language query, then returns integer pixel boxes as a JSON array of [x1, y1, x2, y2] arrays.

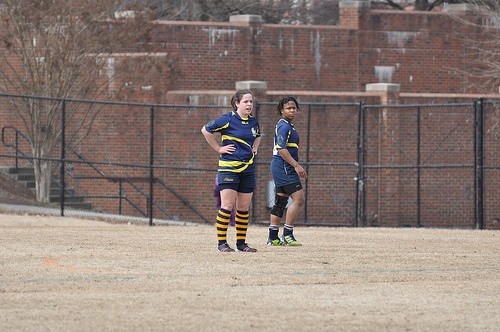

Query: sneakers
[[283, 234, 302, 246], [237, 243, 257, 252], [267, 238, 285, 246], [218, 244, 235, 252]]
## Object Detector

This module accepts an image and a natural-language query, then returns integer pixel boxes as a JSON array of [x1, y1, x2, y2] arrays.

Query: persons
[[201, 89, 261, 253], [267, 97, 306, 247]]
